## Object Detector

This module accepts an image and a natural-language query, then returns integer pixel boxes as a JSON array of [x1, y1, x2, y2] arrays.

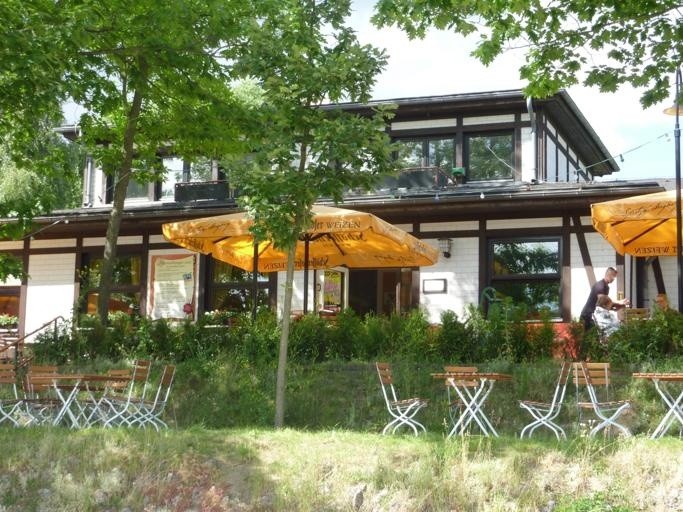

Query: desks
[[632, 372, 683, 441]]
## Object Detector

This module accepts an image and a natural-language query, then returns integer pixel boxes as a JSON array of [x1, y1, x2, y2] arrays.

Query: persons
[[580, 266, 631, 329], [590, 292, 627, 342]]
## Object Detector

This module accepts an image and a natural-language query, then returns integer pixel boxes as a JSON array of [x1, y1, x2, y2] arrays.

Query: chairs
[[374, 359, 633, 443], [0, 338, 177, 435], [591, 312, 608, 345], [624, 308, 650, 327], [290, 305, 340, 329]]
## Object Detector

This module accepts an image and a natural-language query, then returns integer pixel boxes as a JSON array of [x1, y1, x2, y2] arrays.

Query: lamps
[[437, 235, 453, 258]]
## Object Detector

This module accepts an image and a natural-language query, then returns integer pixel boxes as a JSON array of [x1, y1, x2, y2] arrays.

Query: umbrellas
[[587, 186, 683, 258], [159, 200, 438, 313]]
